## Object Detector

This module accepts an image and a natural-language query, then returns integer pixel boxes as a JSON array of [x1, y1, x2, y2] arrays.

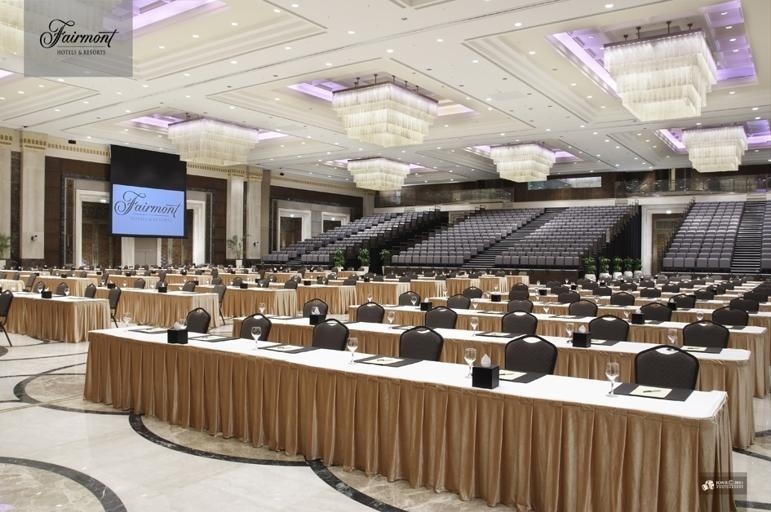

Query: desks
[[78, 324, 737, 512]]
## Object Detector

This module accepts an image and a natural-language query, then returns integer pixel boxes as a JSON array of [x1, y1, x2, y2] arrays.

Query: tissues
[[18, 265, 452, 293]]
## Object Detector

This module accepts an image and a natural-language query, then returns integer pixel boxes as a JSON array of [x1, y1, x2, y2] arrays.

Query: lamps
[[489, 141, 556, 183], [681, 123, 748, 173], [332, 73, 437, 148], [604, 19, 719, 123], [166, 118, 260, 168], [348, 156, 410, 191]]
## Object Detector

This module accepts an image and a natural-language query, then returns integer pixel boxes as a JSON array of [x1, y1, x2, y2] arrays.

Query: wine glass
[[123, 313, 133, 334], [666, 328, 679, 352], [252, 327, 262, 349], [258, 303, 267, 319], [0, 252, 358, 301], [347, 337, 359, 366], [606, 363, 621, 396], [312, 305, 320, 315], [465, 347, 478, 377], [366, 270, 705, 338], [177, 319, 188, 329]]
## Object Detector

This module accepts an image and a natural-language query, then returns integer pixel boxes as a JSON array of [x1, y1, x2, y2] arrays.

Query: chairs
[[508, 273, 771, 390]]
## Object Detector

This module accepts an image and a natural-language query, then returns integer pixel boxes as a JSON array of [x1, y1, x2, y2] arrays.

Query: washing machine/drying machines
[[471, 352, 501, 390]]
